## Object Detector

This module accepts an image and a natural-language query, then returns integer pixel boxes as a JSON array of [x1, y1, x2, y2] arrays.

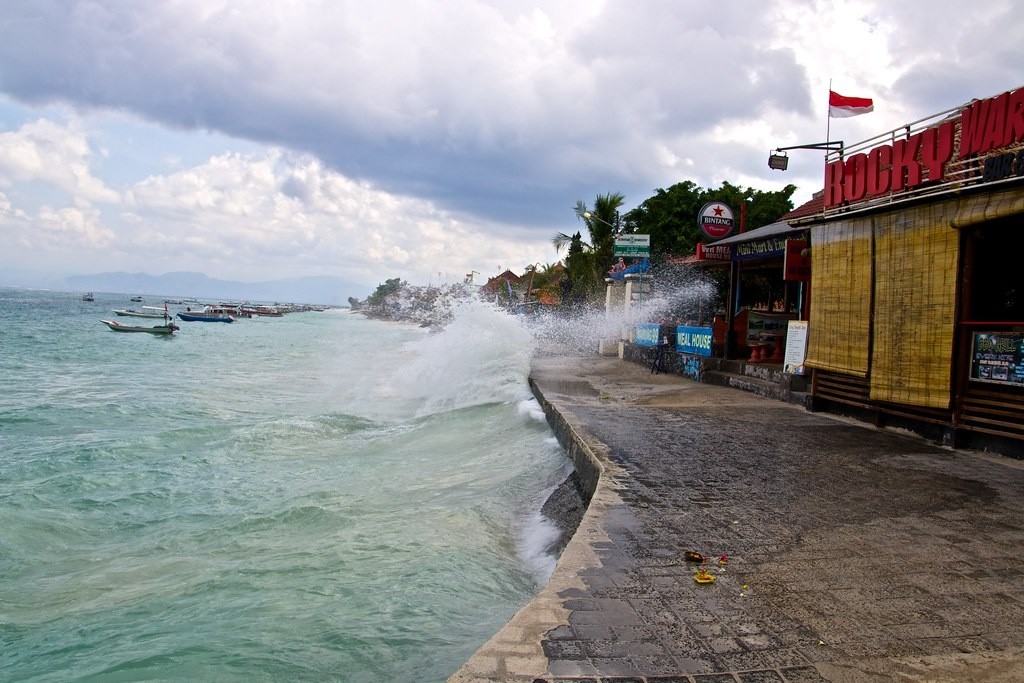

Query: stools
[[748, 340, 771, 365]]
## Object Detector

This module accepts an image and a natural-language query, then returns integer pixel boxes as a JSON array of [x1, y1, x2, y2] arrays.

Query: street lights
[[582, 210, 623, 234]]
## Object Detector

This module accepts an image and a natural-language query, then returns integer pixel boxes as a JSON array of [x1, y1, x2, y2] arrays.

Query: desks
[[761, 332, 786, 362]]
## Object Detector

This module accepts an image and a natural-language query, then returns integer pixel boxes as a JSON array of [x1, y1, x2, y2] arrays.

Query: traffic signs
[[614, 232, 650, 258]]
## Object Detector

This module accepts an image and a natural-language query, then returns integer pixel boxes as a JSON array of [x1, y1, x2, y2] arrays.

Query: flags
[[827, 90, 873, 119]]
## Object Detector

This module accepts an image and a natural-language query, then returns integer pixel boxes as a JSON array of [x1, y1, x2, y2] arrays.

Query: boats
[[163, 298, 181, 305], [201, 301, 331, 319], [177, 312, 234, 323], [83, 291, 95, 301], [112, 306, 170, 320], [183, 299, 199, 303], [130, 297, 145, 302], [99, 302, 180, 334]]
